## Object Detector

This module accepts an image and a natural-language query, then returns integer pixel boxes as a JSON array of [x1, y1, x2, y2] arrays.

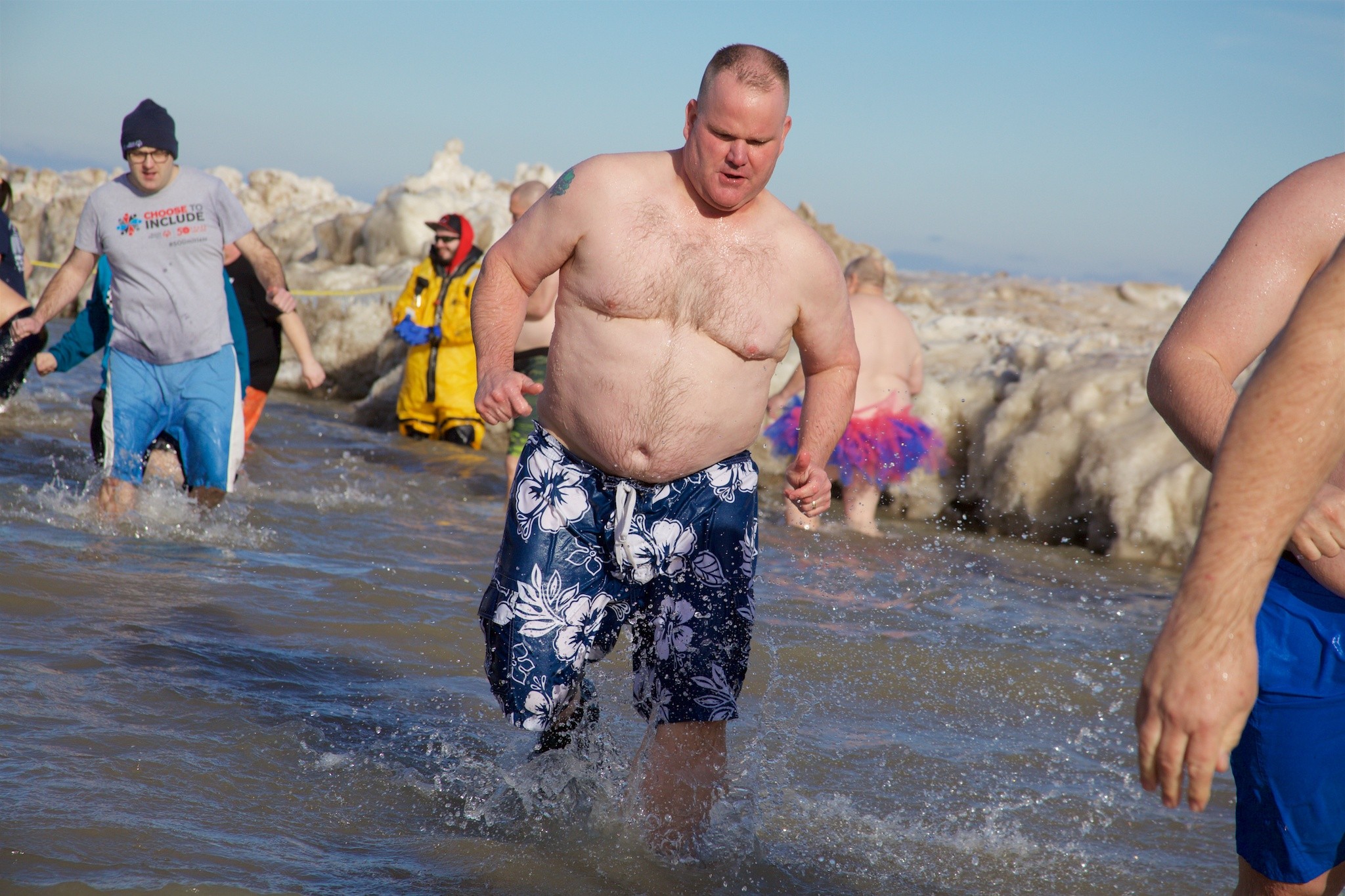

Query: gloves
[[410, 326, 439, 345], [394, 314, 415, 342]]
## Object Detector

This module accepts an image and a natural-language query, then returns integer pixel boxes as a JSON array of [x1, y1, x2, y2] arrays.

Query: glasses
[[125, 148, 170, 164], [434, 235, 460, 243]]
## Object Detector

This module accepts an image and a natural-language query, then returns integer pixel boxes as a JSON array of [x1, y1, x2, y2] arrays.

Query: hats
[[425, 214, 460, 233], [121, 99, 178, 161]]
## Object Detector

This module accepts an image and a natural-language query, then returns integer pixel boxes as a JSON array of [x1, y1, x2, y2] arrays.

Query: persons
[[33, 250, 248, 489], [1, 177, 48, 413], [505, 181, 559, 499], [1147, 153, 1345, 896], [391, 214, 488, 450], [470, 44, 860, 854], [8, 98, 297, 518], [217, 243, 326, 486], [761, 255, 946, 536], [1133, 239, 1343, 810]]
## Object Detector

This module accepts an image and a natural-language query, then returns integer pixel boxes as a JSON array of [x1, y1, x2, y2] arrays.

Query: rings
[[812, 501, 816, 509]]
